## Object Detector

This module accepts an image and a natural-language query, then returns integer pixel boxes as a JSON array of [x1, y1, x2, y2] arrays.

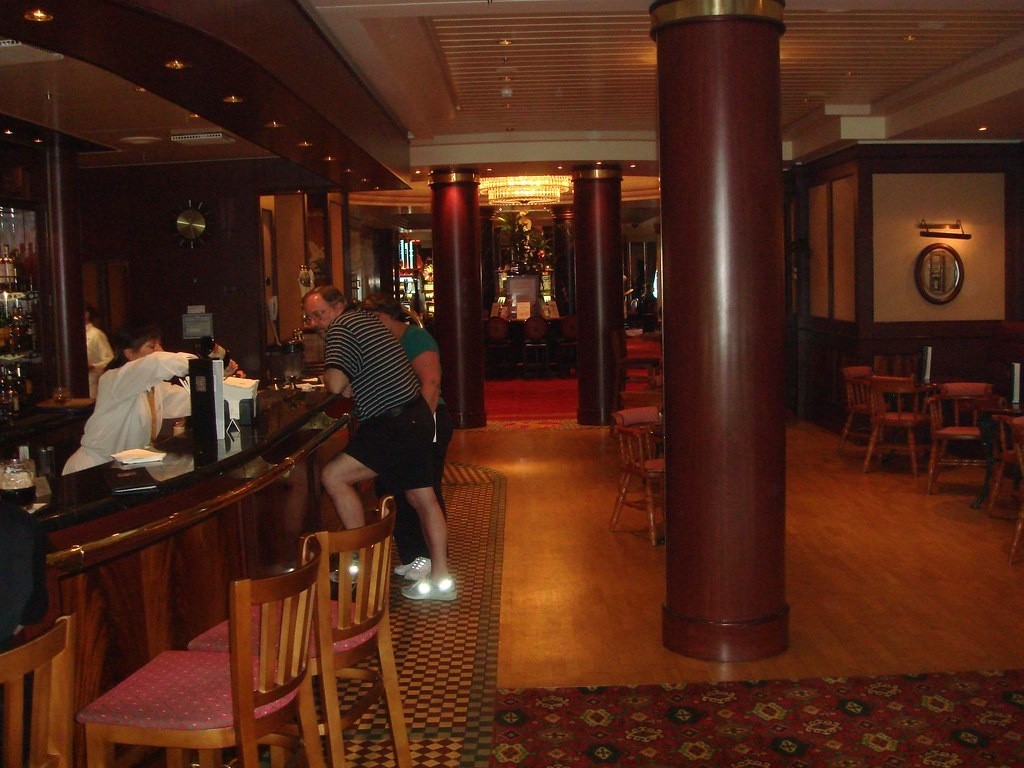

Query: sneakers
[[328, 560, 359, 584], [404, 556, 432, 582], [400, 577, 458, 601], [394, 558, 418, 575]]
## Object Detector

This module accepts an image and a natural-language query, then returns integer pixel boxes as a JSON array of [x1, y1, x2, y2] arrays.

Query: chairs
[[610, 391, 666, 547], [0, 611, 79, 768], [74, 533, 328, 768], [839, 366, 1024, 562], [611, 333, 659, 394], [185, 494, 416, 768]]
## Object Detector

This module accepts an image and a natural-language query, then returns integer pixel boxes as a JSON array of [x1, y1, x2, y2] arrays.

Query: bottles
[[195, 336, 241, 377], [0, 358, 33, 418], [0, 293, 38, 357], [0, 242, 37, 292]]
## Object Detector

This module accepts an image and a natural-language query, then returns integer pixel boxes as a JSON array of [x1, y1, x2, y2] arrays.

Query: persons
[[62, 319, 245, 476], [643, 283, 658, 311], [556, 284, 573, 314], [83, 299, 117, 370], [301, 284, 457, 600]]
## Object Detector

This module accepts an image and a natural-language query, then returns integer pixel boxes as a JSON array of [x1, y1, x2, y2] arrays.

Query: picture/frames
[[915, 242, 965, 305]]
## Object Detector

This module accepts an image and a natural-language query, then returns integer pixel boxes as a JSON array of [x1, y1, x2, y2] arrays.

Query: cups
[[51, 387, 70, 406]]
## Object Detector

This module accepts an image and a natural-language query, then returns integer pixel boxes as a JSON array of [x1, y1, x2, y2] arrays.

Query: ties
[[146, 387, 157, 448]]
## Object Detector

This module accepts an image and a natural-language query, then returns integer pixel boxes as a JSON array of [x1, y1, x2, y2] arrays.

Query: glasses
[[306, 306, 329, 323]]
